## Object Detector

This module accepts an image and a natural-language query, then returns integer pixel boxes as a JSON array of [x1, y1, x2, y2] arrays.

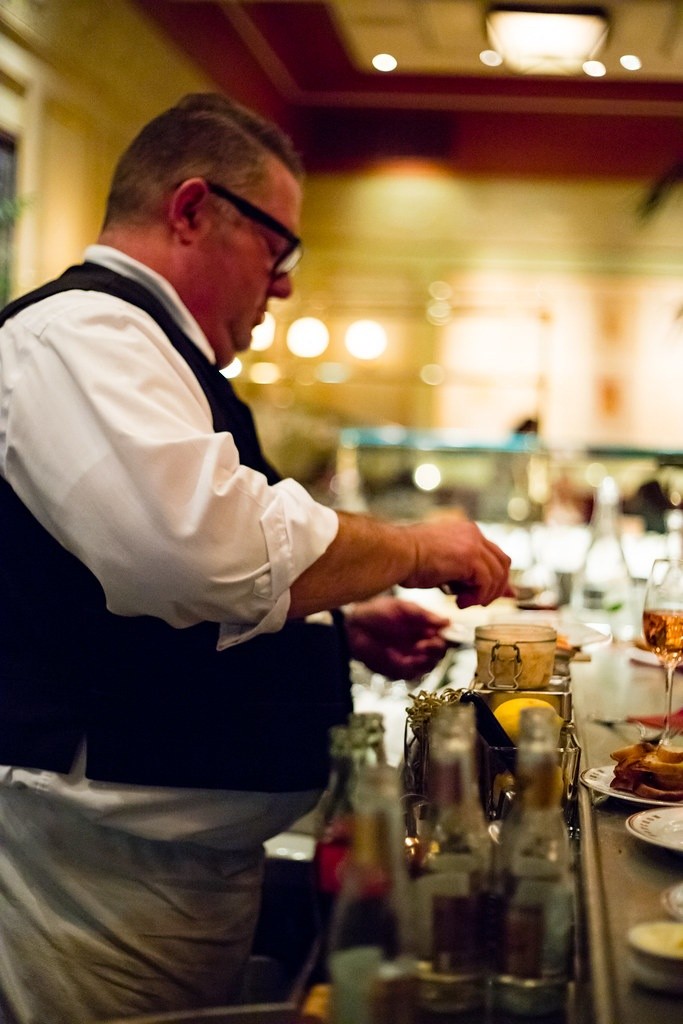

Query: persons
[[0, 89, 512, 1023]]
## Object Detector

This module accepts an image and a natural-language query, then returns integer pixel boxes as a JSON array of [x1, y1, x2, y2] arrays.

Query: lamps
[[483, 0, 609, 79]]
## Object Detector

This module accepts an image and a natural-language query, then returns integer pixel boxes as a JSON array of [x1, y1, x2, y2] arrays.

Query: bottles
[[408, 704, 488, 1013], [315, 714, 398, 985], [491, 708, 571, 1015], [583, 478, 631, 640], [330, 766, 418, 1024]]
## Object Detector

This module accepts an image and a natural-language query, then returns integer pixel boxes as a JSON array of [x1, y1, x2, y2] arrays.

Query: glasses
[[172, 178, 303, 276]]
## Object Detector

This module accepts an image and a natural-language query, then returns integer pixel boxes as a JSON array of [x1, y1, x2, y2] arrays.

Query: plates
[[625, 807, 683, 853], [661, 882, 683, 922], [580, 765, 683, 807], [440, 616, 605, 648]]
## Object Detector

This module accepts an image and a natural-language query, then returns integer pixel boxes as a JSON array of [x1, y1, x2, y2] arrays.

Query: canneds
[[474, 625, 560, 691]]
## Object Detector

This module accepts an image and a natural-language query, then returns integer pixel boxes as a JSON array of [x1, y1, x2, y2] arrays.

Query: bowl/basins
[[626, 923, 683, 991]]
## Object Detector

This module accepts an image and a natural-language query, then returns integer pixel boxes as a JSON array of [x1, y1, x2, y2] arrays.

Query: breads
[[607, 741, 683, 801]]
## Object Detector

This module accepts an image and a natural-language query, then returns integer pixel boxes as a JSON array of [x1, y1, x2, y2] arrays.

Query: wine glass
[[643, 558, 683, 746]]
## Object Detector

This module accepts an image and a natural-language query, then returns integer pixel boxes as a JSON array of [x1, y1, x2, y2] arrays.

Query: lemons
[[489, 698, 563, 747]]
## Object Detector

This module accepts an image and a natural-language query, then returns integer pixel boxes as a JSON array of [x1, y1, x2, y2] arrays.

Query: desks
[[284, 573, 683, 1024]]
